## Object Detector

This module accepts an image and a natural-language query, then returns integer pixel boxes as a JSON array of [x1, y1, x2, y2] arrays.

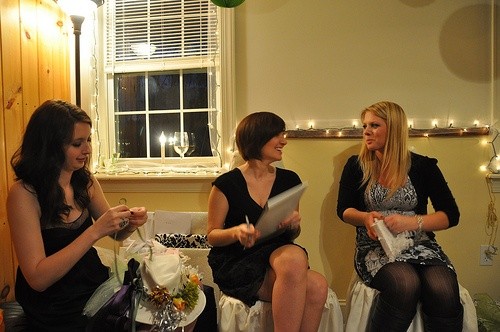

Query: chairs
[[344, 268, 478, 332], [217, 288, 344, 332]]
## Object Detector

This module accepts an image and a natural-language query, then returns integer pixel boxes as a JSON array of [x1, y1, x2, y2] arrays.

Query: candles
[[158, 131, 166, 164]]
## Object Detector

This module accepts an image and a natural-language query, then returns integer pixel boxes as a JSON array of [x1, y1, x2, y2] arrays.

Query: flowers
[[171, 262, 205, 316]]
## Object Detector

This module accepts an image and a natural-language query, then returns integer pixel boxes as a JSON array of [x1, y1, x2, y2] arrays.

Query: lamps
[[57, 0, 104, 109]]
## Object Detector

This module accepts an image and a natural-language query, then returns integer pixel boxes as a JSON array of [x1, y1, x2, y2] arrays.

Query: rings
[[119, 219, 129, 229]]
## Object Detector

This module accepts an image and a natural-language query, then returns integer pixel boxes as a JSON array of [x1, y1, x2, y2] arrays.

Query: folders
[[254, 183, 307, 239]]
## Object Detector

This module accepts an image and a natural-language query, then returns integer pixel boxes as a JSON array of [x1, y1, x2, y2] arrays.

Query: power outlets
[[480, 245, 493, 266]]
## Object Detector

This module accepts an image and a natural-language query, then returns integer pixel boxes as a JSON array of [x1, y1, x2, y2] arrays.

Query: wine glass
[[172, 131, 189, 171], [184, 132, 196, 157]]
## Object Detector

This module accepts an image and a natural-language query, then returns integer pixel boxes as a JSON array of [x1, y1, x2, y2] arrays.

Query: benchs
[[119, 210, 215, 286]]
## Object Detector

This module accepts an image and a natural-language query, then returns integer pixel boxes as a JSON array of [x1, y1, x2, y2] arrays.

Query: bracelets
[[414, 214, 423, 233]]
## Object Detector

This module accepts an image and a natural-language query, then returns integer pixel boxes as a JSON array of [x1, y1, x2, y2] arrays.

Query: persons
[[6, 100, 149, 332], [336, 101, 460, 332], [206, 111, 329, 332]]
